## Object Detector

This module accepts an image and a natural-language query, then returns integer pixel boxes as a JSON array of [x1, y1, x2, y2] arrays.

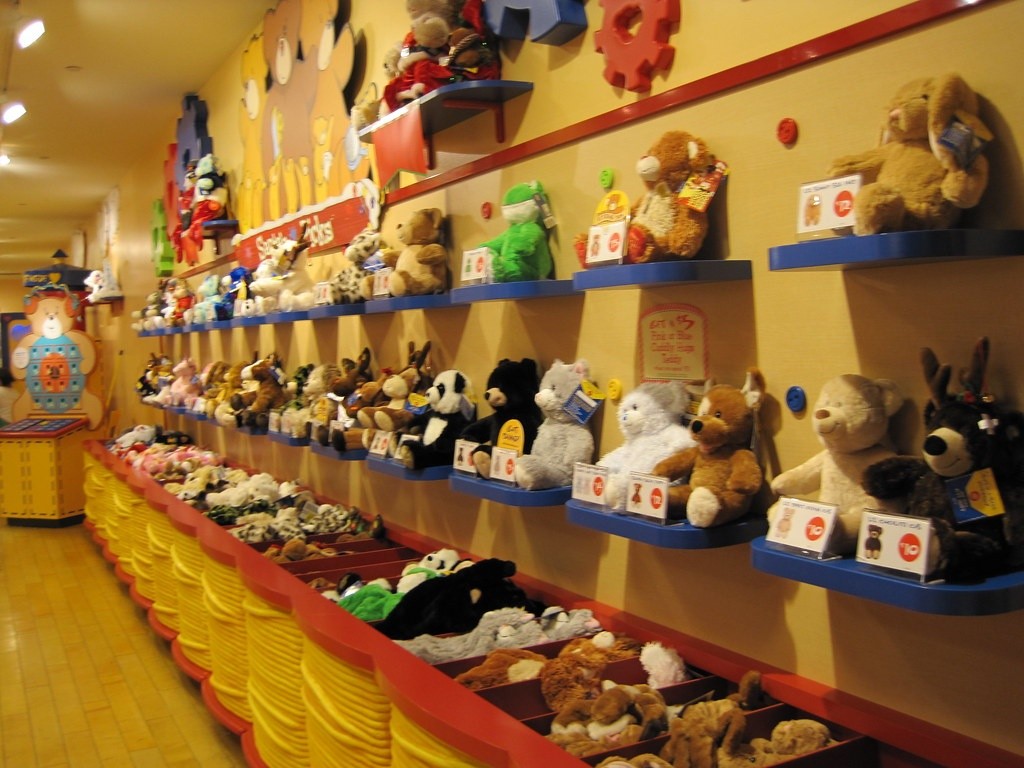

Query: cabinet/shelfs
[[132, 214, 1024, 613]]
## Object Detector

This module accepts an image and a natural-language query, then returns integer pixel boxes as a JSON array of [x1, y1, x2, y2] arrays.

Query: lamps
[[13, 13, 45, 48], [0, 92, 27, 126]]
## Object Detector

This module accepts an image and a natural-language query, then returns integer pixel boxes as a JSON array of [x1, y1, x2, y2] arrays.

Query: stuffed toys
[[595, 367, 766, 528], [771, 335, 1024, 583], [103, 423, 388, 563], [462, 356, 594, 491], [135, 347, 478, 471], [573, 130, 714, 267], [477, 180, 557, 281], [184, 222, 317, 324], [350, 0, 496, 131], [320, 546, 832, 768], [359, 207, 448, 297], [825, 71, 994, 237], [331, 228, 387, 304], [131, 277, 197, 331]]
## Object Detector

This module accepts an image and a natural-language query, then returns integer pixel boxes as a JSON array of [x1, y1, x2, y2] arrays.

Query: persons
[[169, 153, 228, 266]]
